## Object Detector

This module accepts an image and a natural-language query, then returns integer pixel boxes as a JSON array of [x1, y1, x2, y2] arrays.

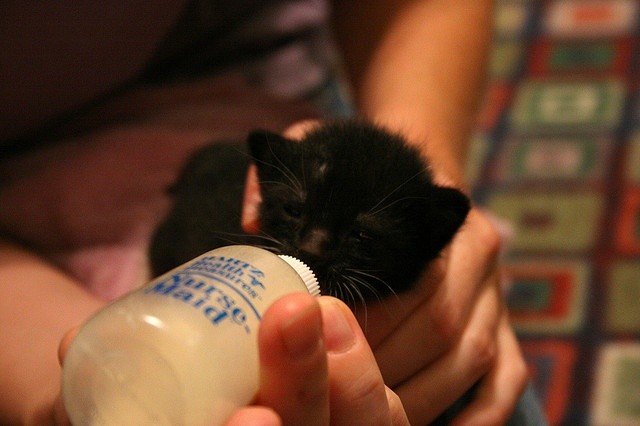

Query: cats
[[148, 114, 472, 330]]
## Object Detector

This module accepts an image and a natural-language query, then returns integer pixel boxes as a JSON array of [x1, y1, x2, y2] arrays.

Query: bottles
[[64, 245, 320, 426]]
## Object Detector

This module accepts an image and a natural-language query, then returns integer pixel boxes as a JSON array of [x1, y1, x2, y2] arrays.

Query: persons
[[0, 0, 529, 426]]
